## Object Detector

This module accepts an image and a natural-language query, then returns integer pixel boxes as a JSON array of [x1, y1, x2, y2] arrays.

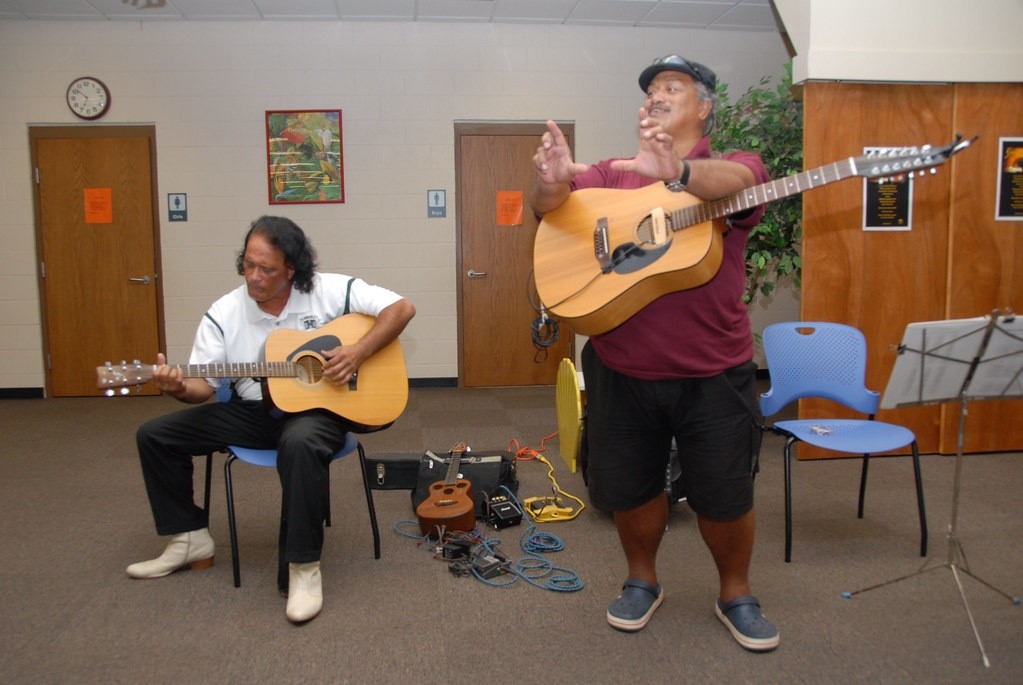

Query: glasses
[[652, 54, 704, 83]]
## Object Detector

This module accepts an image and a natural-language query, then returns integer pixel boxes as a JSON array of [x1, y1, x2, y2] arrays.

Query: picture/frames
[[265, 109, 346, 206]]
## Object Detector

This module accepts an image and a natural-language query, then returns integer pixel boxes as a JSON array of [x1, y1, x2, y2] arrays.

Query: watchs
[[663, 159, 690, 194]]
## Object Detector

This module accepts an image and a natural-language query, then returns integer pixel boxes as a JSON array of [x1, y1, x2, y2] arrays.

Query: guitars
[[96, 312, 409, 434], [534, 134, 979, 337], [416, 442, 476, 537]]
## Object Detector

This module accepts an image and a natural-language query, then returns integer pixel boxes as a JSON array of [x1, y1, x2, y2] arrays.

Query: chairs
[[751, 320, 928, 566], [198, 374, 382, 599]]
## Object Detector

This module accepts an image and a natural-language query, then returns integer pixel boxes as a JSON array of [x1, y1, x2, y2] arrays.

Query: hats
[[638, 62, 718, 95]]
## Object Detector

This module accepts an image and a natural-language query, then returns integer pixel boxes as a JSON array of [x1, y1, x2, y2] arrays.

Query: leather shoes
[[284, 561, 325, 625], [126, 528, 217, 580]]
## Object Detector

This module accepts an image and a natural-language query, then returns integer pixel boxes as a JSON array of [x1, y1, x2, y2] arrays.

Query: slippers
[[715, 596, 780, 651], [606, 577, 665, 633]]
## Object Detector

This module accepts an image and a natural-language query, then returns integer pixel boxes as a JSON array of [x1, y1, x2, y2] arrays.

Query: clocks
[[66, 77, 112, 120]]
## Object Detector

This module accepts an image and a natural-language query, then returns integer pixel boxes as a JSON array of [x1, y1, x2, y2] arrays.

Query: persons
[[527, 54, 780, 654], [125, 215, 417, 625]]
